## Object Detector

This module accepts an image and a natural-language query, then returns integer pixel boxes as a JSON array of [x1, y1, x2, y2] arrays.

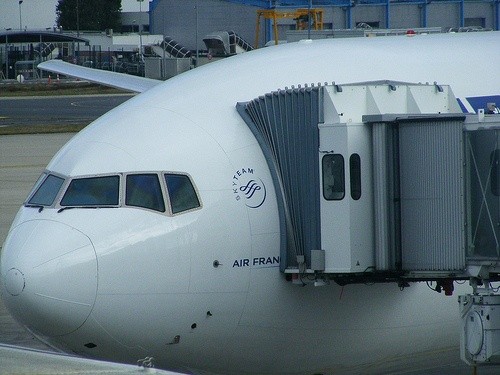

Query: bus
[[15, 60, 45, 78]]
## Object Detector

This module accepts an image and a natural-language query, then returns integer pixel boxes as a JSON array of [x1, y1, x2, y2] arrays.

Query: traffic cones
[[56, 74, 61, 82], [48, 75, 52, 86], [76, 77, 81, 81]]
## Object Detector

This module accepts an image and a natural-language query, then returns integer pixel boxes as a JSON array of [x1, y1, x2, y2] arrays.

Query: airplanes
[[0, 31, 499, 375]]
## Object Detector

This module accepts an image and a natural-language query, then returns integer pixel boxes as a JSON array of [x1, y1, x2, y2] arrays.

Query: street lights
[[132, 20, 135, 33], [5, 29, 11, 80], [19, 0, 24, 54], [137, 0, 144, 65]]
